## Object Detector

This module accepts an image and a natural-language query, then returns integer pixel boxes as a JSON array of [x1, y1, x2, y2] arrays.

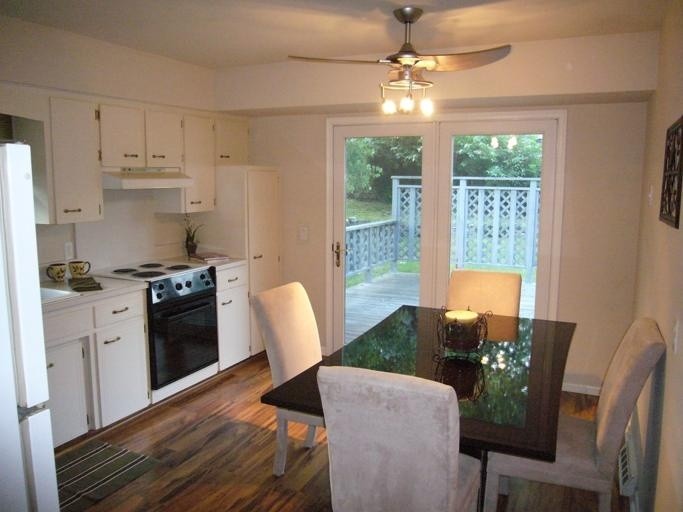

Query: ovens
[[150, 294, 220, 389]]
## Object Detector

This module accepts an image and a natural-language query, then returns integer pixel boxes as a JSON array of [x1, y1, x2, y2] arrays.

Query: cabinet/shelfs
[[216, 254, 251, 370], [183, 106, 249, 213], [186, 169, 286, 357], [0, 81, 103, 225], [42, 278, 149, 447], [100, 98, 183, 170]]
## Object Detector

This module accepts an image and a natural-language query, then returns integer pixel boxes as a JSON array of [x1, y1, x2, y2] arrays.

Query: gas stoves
[[92, 260, 216, 312]]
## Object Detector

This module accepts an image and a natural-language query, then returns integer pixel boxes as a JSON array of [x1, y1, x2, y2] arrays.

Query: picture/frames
[[658, 115, 683, 228]]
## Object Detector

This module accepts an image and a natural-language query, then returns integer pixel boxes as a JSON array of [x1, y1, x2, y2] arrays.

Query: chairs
[[253, 282, 322, 477], [482, 319, 665, 512], [447, 270, 522, 318], [317, 365, 483, 512]]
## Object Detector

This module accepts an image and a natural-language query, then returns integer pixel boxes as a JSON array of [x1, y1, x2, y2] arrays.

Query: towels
[[69, 278, 102, 291]]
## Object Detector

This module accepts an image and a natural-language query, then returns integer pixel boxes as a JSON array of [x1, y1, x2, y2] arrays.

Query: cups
[[44, 263, 66, 282], [68, 260, 91, 278]]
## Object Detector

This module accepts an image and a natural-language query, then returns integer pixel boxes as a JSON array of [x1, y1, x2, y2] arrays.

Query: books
[[190, 253, 229, 263]]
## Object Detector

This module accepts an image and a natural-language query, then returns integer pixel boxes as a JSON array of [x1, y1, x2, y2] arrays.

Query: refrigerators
[[0, 140, 61, 509]]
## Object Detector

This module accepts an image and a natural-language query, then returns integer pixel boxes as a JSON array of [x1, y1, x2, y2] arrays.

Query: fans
[[287, 6, 510, 70]]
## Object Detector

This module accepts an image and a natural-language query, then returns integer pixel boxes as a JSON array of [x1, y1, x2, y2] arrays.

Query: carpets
[[55, 435, 159, 512]]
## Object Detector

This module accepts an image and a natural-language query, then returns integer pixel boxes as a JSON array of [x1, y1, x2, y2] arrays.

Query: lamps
[[380, 72, 436, 114]]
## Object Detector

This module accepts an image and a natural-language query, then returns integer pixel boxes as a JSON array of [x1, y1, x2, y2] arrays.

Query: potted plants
[[185, 223, 203, 256]]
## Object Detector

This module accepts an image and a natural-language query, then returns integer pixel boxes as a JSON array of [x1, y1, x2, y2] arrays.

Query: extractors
[[100, 165, 200, 191]]
